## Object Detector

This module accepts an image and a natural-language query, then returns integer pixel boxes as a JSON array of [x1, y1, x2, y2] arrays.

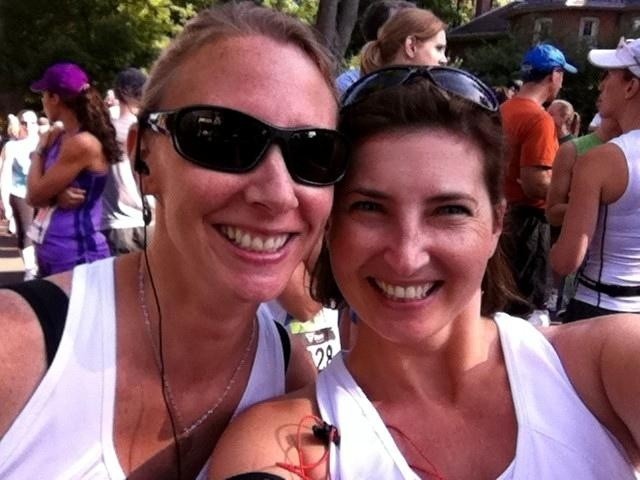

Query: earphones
[[129, 140, 153, 176]]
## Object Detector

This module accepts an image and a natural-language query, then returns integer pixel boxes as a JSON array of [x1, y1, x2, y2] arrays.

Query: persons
[[0, 1, 639, 480]]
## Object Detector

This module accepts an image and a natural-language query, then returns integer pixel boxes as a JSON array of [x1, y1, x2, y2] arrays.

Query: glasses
[[616, 37, 640, 66], [141, 102, 349, 188], [341, 63, 500, 116]]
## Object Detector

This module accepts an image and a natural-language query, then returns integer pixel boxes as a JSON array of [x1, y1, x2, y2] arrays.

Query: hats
[[30, 64, 89, 93], [521, 44, 577, 74], [589, 38, 640, 76]]
